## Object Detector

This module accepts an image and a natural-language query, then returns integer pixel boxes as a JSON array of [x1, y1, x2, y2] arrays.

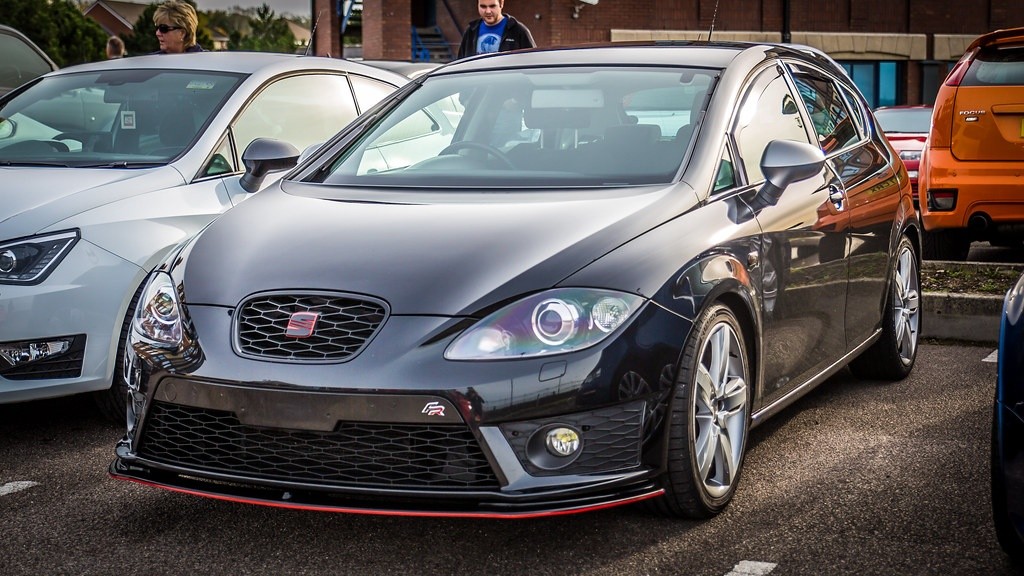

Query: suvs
[[918, 25, 1024, 261]]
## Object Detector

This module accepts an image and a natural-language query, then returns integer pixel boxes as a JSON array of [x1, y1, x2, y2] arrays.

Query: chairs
[[113, 102, 195, 155], [669, 87, 708, 172], [504, 92, 591, 170]]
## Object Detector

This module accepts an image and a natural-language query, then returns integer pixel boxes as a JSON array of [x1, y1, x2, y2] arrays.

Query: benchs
[[577, 123, 678, 172]]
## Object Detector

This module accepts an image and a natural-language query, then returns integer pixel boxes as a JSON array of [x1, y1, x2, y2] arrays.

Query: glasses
[[154, 23, 184, 34]]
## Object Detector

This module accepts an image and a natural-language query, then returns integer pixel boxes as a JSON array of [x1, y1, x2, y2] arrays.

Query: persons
[[457, 0, 537, 60], [152, 0, 203, 54], [106, 36, 125, 59]]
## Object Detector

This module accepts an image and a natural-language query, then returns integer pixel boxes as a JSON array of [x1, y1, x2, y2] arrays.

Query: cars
[[0, 19, 711, 438], [990, 272, 1024, 561], [108, 37, 927, 526], [870, 104, 936, 202]]
